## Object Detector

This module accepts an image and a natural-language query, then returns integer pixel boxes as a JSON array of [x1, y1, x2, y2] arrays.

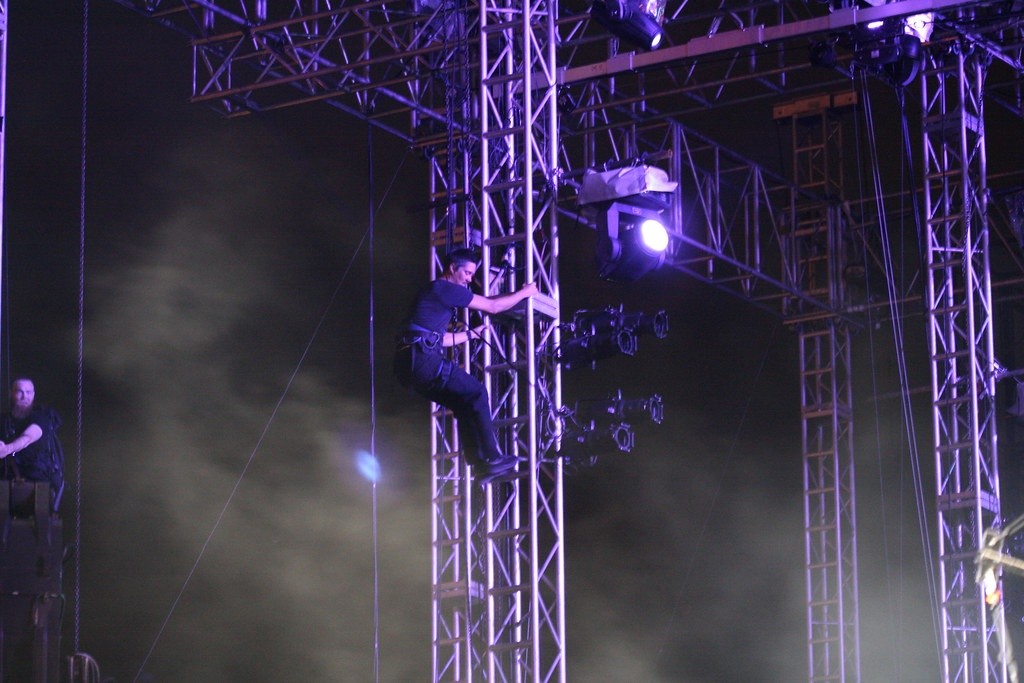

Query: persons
[[396, 247, 538, 487], [0, 374, 64, 511]]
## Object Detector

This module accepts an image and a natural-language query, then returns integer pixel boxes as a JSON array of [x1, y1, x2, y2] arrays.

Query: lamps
[[594, 200, 669, 272], [559, 301, 671, 477]]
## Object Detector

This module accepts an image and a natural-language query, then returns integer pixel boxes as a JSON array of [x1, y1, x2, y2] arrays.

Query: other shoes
[[475, 470, 507, 486], [486, 454, 518, 475]]
